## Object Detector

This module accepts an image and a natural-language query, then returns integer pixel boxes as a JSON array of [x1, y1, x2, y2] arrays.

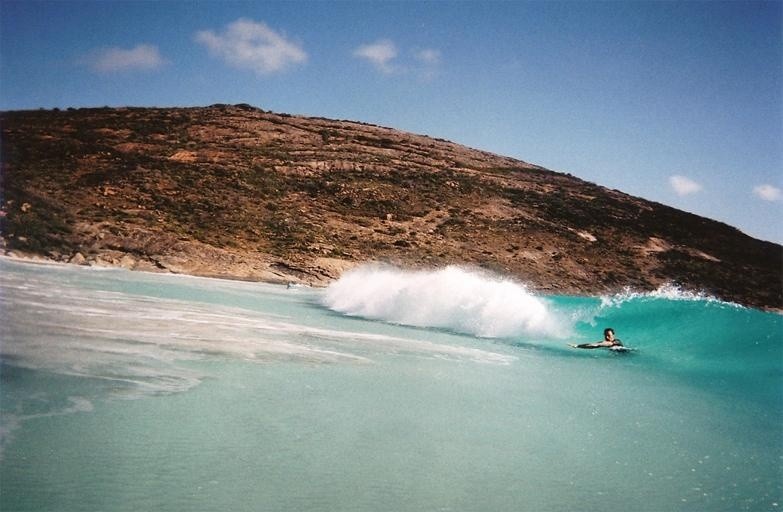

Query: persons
[[575, 327, 625, 349]]
